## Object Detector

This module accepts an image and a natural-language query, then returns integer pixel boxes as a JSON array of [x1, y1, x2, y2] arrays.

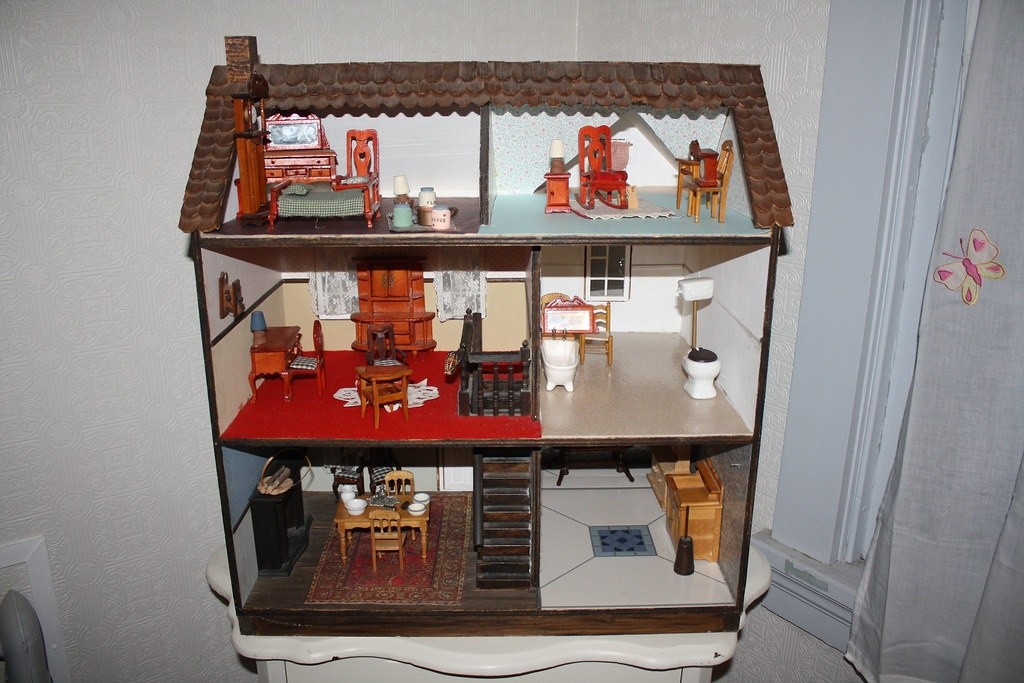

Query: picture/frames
[[264, 119, 322, 152]]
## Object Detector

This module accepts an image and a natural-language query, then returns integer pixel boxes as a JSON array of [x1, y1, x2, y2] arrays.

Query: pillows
[[281, 181, 314, 195]]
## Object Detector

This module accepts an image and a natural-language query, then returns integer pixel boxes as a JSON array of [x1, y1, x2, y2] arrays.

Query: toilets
[[681, 347, 720, 399]]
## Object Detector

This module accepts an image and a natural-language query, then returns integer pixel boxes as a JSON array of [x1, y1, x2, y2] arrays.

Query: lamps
[[251, 311, 267, 345], [549, 140, 564, 173]]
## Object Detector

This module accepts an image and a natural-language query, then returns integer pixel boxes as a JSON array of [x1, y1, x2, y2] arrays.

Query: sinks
[[541, 339, 580, 392]]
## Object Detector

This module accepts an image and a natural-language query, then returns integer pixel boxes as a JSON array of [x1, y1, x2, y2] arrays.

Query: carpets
[[302, 491, 472, 606], [334, 378, 440, 413], [569, 196, 676, 221]]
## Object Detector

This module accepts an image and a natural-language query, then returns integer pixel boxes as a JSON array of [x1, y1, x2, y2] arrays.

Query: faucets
[[562, 328, 567, 339], [552, 328, 556, 339]]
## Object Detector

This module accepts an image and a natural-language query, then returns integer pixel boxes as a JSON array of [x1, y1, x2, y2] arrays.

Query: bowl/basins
[[344, 499, 367, 516], [412, 492, 431, 505], [407, 503, 426, 517]]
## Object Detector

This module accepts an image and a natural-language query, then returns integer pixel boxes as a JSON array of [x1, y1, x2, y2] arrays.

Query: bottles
[[431, 204, 450, 230], [341, 485, 355, 506], [418, 187, 437, 207], [394, 203, 413, 227]]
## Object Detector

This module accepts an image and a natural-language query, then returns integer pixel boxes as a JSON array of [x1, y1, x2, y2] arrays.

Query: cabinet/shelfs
[[263, 149, 340, 183], [179, 31, 795, 683], [249, 326, 303, 404], [350, 256, 439, 360], [646, 445, 724, 563], [543, 172, 571, 214]]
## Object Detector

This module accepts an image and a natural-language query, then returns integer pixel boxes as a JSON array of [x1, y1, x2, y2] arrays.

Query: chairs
[[541, 294, 613, 367], [688, 139, 734, 223], [365, 323, 415, 384], [331, 447, 400, 503], [577, 126, 628, 210], [288, 320, 326, 398], [386, 470, 416, 540], [336, 129, 379, 191], [368, 509, 407, 572]]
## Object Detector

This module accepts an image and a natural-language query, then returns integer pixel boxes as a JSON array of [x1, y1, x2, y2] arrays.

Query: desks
[[332, 495, 430, 565], [674, 156, 719, 217], [355, 365, 412, 430]]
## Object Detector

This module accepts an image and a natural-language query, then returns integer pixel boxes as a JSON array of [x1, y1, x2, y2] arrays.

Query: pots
[[414, 206, 458, 224]]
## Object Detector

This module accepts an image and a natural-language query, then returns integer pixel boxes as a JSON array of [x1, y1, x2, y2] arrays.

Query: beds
[[269, 179, 381, 230]]
